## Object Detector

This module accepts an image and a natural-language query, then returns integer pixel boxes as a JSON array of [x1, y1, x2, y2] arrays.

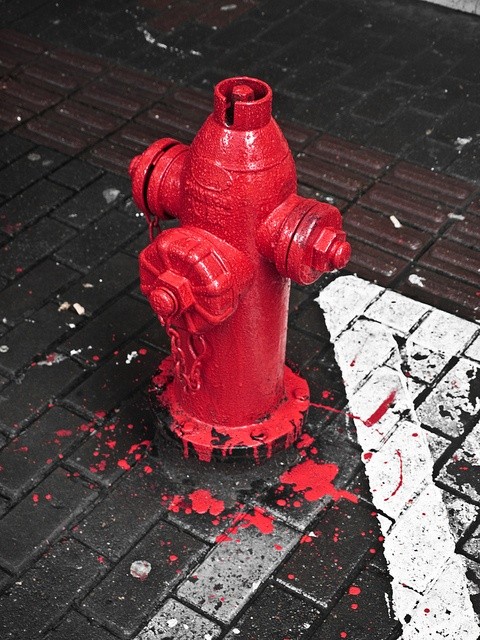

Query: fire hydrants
[[128, 76, 352, 462]]
